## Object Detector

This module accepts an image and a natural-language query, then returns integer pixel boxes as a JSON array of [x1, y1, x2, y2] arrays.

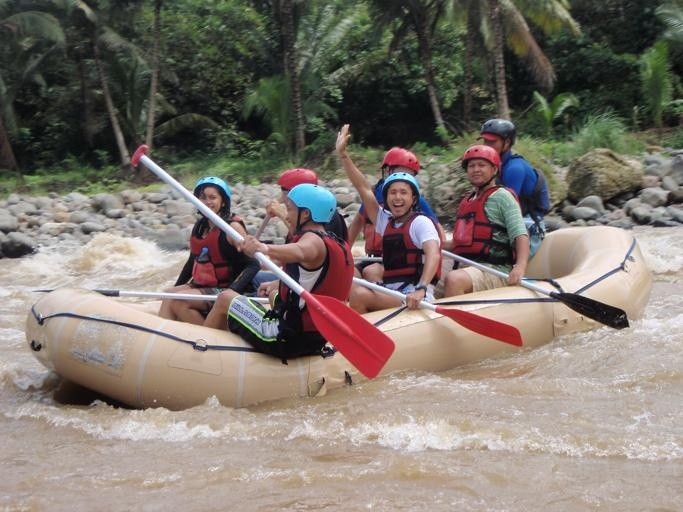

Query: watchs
[[413, 283, 427, 292]]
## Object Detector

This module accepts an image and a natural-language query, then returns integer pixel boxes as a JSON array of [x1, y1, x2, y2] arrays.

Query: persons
[[202, 186, 353, 357], [250, 166, 352, 292], [337, 123, 441, 315], [478, 120, 551, 259], [159, 177, 251, 326], [441, 144, 529, 298], [348, 147, 445, 283]]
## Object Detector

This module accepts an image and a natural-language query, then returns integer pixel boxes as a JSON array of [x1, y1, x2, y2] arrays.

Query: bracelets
[[261, 243, 268, 256]]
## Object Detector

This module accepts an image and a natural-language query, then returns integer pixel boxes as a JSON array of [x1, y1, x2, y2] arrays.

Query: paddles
[[441, 249, 628, 329], [353, 277, 524, 348], [33, 288, 272, 304], [131, 145, 396, 381]]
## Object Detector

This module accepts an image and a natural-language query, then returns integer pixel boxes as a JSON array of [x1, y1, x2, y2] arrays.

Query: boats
[[24, 224, 652, 411]]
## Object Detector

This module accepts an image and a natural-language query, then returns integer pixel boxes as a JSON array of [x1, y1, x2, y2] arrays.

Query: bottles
[[192, 247, 210, 288]]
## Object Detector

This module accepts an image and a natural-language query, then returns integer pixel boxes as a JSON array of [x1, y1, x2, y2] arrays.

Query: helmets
[[278, 168, 318, 190], [193, 176, 232, 201], [379, 146, 419, 176], [286, 183, 338, 225], [382, 172, 421, 204], [476, 118, 516, 146], [461, 144, 502, 172]]
